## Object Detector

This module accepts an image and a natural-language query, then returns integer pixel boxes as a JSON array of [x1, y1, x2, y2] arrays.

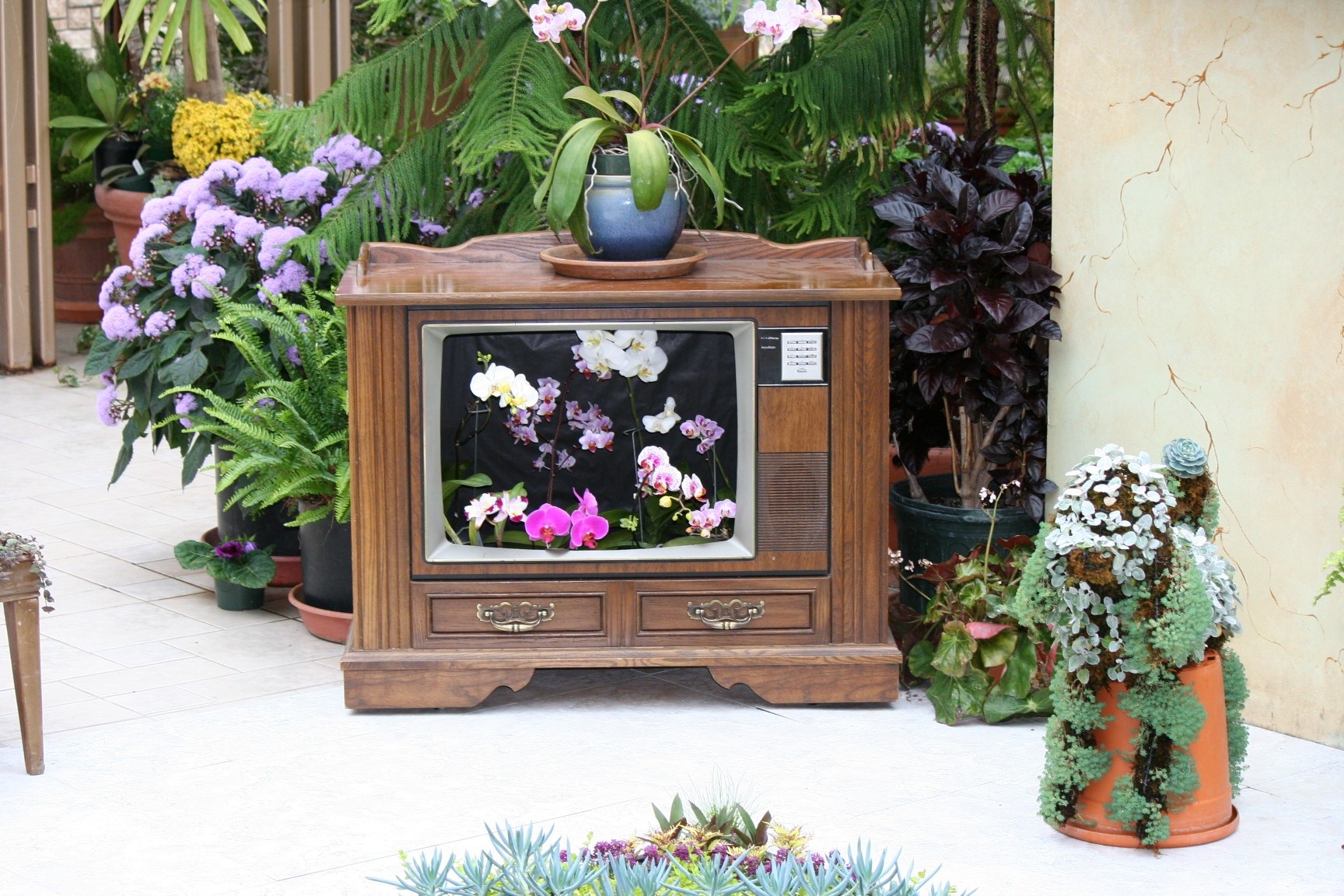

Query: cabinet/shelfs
[[333, 233, 905, 712]]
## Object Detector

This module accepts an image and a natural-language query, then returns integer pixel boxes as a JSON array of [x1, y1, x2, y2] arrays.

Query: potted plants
[[146, 274, 357, 614], [872, 107, 1065, 619]]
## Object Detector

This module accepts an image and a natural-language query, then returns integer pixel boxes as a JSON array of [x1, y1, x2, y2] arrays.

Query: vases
[[1046, 644, 1242, 849], [572, 149, 692, 261], [213, 574, 266, 610], [93, 183, 192, 282], [209, 430, 300, 560]]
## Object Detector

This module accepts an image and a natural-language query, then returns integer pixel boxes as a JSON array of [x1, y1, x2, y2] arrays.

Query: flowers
[[881, 478, 1057, 726], [45, 63, 493, 490], [508, 0, 842, 256], [173, 530, 281, 590], [1007, 435, 1249, 851]]
